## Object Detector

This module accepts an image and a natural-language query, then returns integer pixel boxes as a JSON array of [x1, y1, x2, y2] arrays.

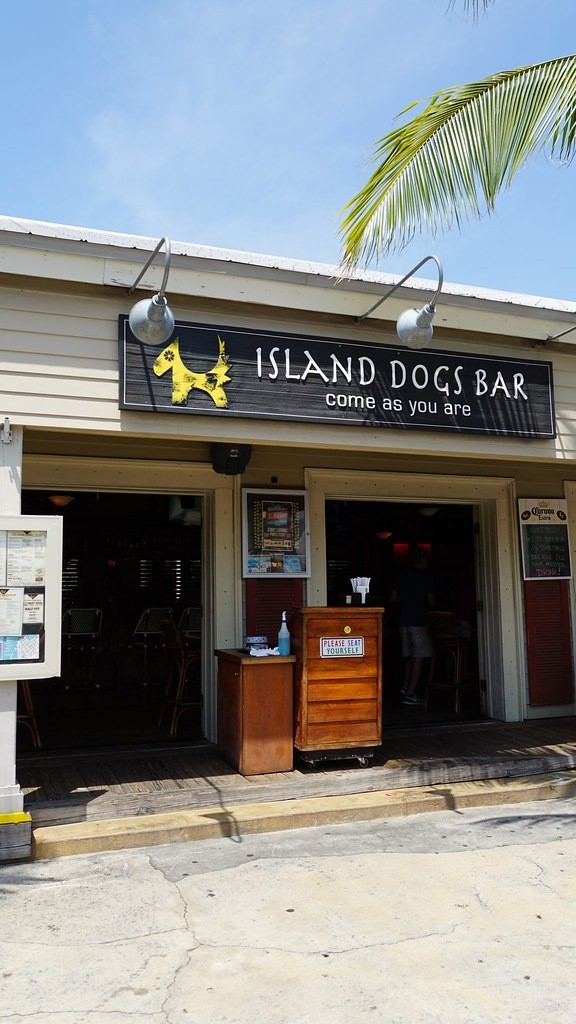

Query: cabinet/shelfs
[[294, 604, 384, 771], [215, 648, 297, 776]]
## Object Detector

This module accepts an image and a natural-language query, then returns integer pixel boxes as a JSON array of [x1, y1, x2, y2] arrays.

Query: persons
[[390, 548, 436, 705]]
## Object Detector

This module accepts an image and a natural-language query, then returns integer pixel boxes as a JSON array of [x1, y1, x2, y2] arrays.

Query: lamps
[[355, 254, 444, 350], [418, 506, 439, 518], [128, 235, 177, 348], [46, 493, 80, 507], [376, 525, 393, 541]]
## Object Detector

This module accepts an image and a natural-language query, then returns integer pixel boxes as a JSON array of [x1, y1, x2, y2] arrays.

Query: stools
[[62, 608, 103, 711], [17, 680, 42, 749], [168, 607, 204, 709], [130, 607, 174, 707], [159, 617, 203, 736], [423, 610, 477, 712]]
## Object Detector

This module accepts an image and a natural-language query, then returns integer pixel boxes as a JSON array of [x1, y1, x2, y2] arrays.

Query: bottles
[[277, 610, 292, 656]]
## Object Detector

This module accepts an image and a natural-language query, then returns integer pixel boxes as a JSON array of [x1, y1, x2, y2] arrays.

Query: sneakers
[[403, 694, 424, 705], [400, 687, 407, 695]]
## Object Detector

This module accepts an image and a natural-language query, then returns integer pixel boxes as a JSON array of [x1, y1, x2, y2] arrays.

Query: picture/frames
[[517, 496, 573, 581], [242, 488, 312, 579]]
